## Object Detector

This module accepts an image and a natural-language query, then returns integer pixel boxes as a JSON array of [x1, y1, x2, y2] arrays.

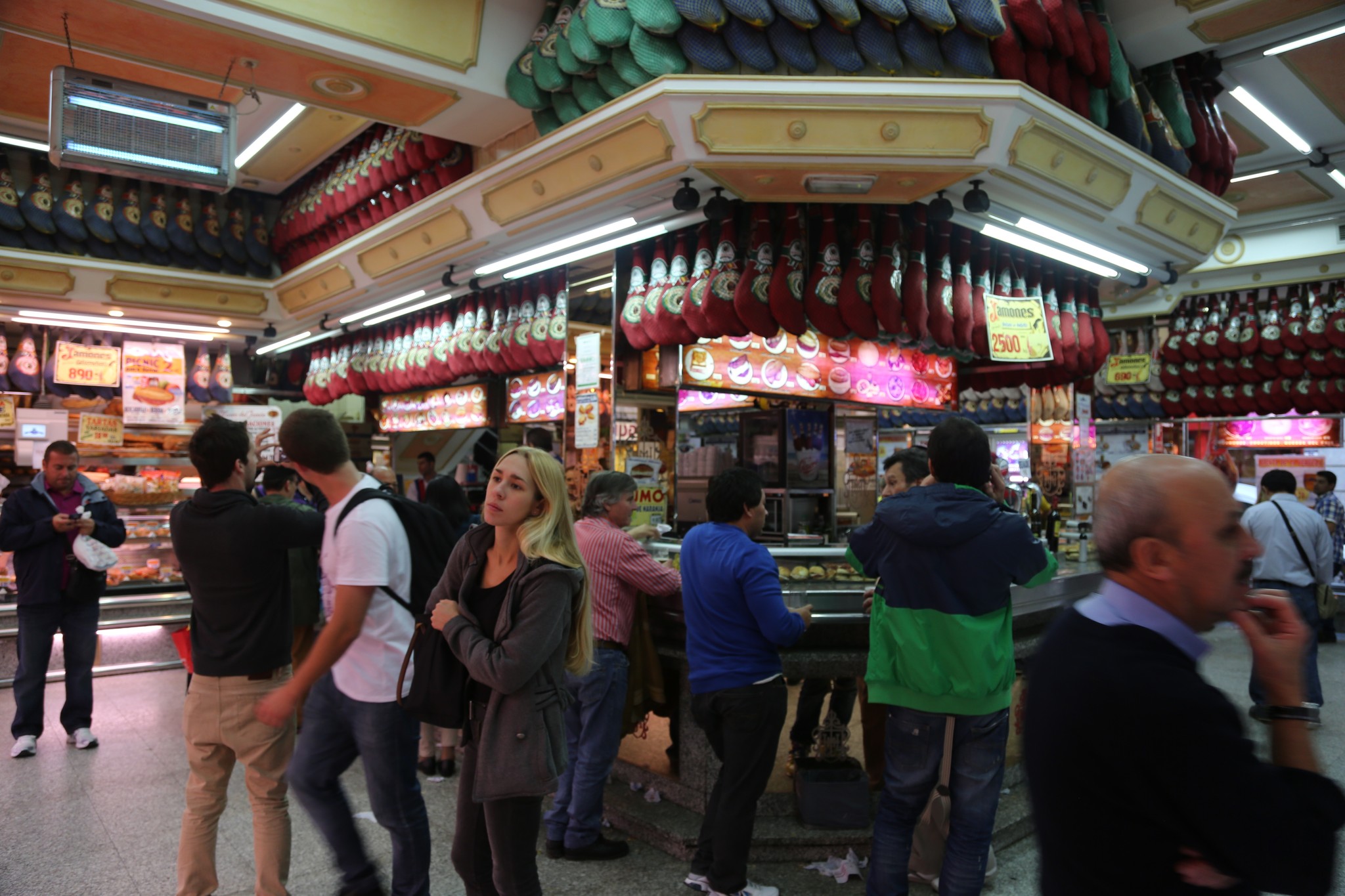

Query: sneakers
[[684, 871, 711, 892], [707, 877, 779, 896], [66, 727, 99, 750], [10, 734, 38, 757]]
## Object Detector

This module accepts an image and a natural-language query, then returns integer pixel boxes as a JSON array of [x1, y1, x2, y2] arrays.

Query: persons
[[424, 445, 597, 896], [1312, 471, 1345, 644], [1240, 469, 1342, 730], [862, 446, 998, 890], [1020, 454, 1345, 896], [682, 467, 813, 896], [256, 408, 473, 896], [844, 416, 1059, 896], [417, 475, 470, 778], [542, 470, 682, 862], [256, 466, 320, 735], [170, 414, 326, 896], [0, 441, 126, 758]]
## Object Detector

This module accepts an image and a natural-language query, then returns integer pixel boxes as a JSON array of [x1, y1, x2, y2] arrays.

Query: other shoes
[[433, 758, 455, 778], [563, 832, 630, 861], [545, 836, 566, 860], [416, 757, 436, 775]]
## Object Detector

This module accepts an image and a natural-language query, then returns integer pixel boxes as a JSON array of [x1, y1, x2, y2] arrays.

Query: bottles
[[1046, 496, 1061, 553], [1028, 492, 1041, 538], [1078, 528, 1087, 562]]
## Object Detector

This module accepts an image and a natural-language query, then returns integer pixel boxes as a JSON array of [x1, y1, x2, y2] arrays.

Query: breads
[[1059, 543, 1096, 559]]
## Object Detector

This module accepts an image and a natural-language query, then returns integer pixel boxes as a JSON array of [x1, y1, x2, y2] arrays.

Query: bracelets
[[1248, 702, 1325, 729]]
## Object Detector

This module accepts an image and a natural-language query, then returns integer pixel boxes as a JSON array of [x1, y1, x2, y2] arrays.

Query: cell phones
[[273, 446, 292, 464], [69, 514, 82, 519]]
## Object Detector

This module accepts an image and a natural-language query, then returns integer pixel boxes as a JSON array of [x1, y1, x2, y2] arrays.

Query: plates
[[379, 387, 486, 431], [508, 373, 562, 419], [685, 330, 954, 406]]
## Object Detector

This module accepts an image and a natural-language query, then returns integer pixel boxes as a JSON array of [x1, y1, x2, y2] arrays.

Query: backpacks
[[333, 487, 460, 619]]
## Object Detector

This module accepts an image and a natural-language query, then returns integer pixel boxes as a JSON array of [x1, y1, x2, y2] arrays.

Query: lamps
[[673, 178, 733, 222], [928, 179, 990, 223]]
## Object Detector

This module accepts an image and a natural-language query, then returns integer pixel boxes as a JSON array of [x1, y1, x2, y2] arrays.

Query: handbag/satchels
[[1316, 580, 1338, 620], [396, 614, 470, 729]]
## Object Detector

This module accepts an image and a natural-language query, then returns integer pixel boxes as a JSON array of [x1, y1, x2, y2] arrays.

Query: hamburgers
[[777, 566, 826, 581]]
